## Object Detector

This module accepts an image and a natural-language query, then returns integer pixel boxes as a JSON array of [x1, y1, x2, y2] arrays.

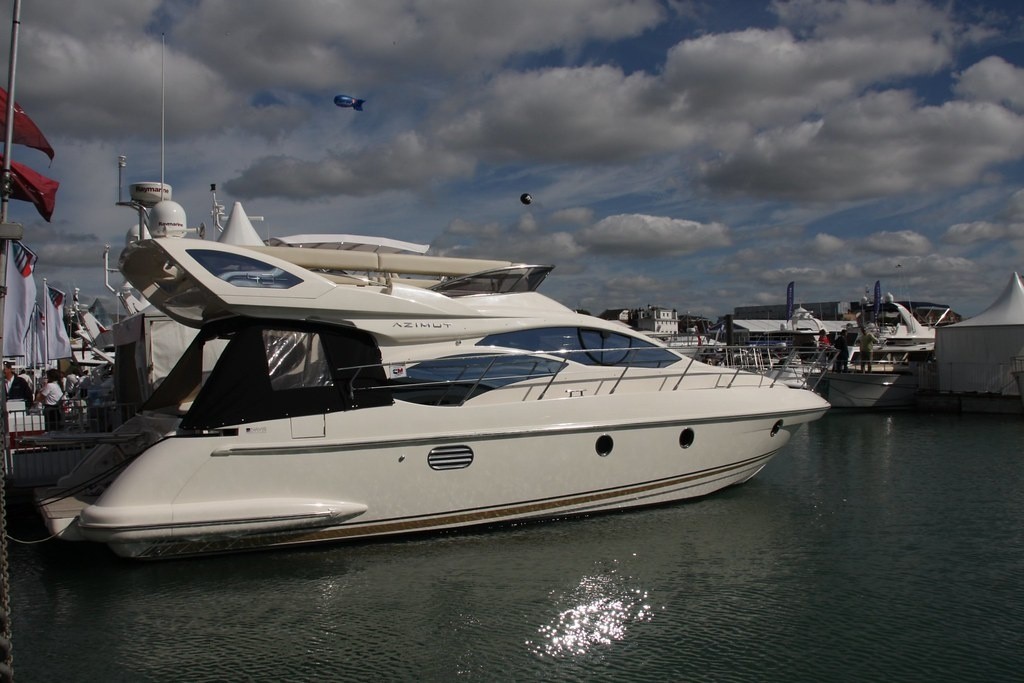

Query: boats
[[707, 292, 960, 409], [0, 33, 832, 556]]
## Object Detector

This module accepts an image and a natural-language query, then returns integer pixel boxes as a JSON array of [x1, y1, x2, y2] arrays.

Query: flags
[[3, 239, 72, 394], [0, 153, 60, 223], [0, 86, 54, 161]]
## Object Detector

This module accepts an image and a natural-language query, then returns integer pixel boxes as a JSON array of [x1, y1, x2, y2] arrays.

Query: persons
[[856, 328, 879, 374], [3, 361, 89, 432], [834, 330, 852, 373], [818, 329, 831, 363]]
